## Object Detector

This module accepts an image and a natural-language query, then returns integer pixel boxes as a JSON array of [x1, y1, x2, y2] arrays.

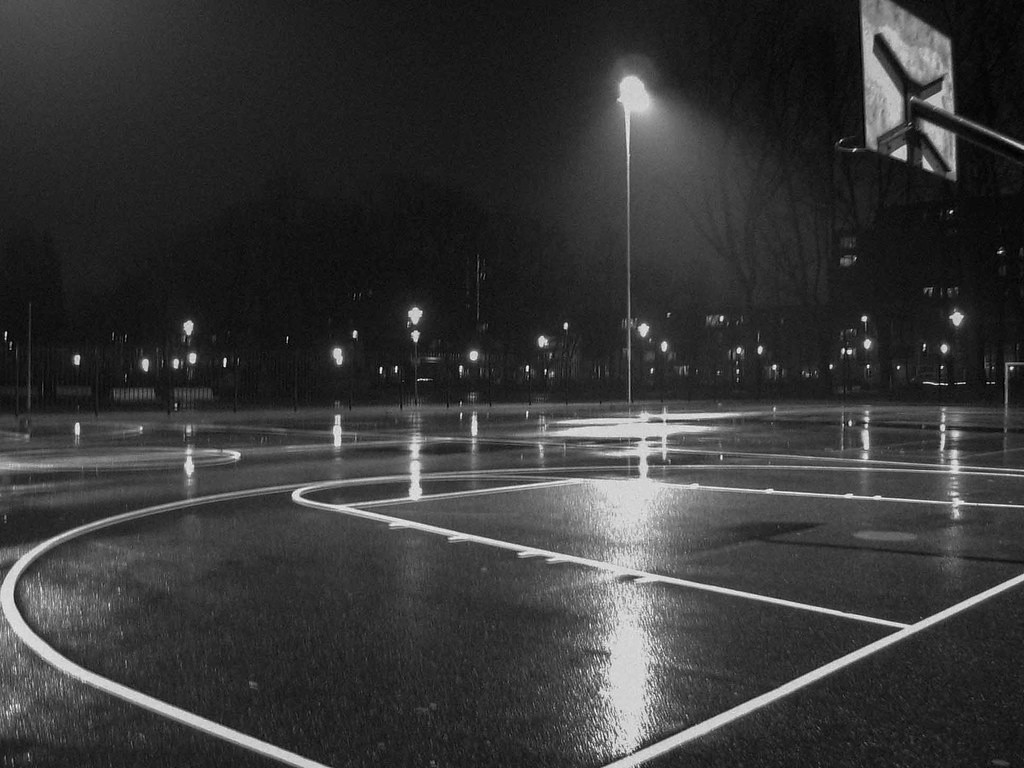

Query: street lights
[[411, 329, 422, 404], [617, 75, 647, 404], [184, 318, 195, 389]]
